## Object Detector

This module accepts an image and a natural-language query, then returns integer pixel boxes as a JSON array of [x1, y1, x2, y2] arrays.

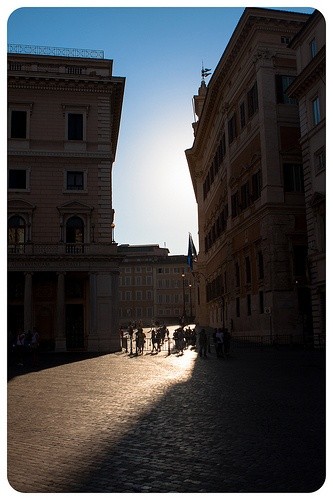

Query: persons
[[197, 328, 209, 359], [120, 315, 200, 354], [13, 326, 42, 365], [211, 327, 231, 360]]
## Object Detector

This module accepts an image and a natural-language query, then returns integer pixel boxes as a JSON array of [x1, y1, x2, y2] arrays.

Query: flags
[[184, 232, 196, 274]]
